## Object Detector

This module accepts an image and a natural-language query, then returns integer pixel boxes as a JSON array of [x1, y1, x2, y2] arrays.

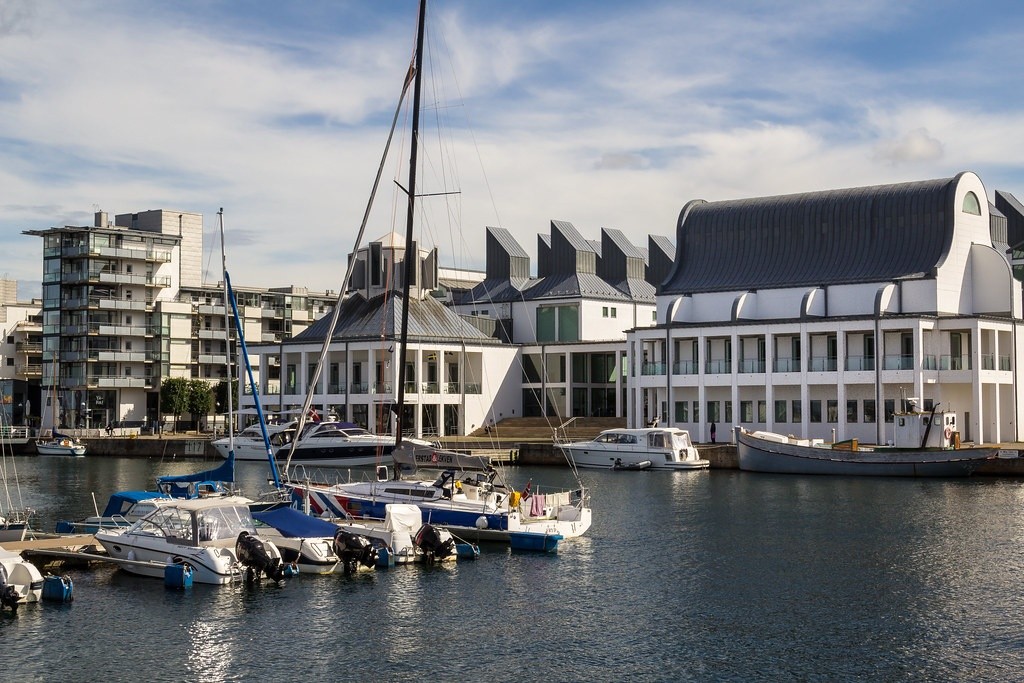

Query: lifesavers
[[945, 427, 952, 439]]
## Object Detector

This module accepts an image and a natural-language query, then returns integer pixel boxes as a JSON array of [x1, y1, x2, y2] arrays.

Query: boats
[[0, 545, 45, 613], [93, 502, 299, 583], [305, 505, 458, 564], [86, 489, 180, 535], [274, 422, 439, 468], [251, 506, 381, 576], [555, 427, 710, 470], [210, 423, 295, 459], [736, 424, 1019, 474]]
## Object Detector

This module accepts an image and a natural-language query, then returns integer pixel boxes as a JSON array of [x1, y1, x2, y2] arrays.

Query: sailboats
[[284, 0, 591, 554], [34, 350, 88, 456]]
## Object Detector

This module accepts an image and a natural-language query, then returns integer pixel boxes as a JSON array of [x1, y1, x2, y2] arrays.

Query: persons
[[710, 420, 716, 443]]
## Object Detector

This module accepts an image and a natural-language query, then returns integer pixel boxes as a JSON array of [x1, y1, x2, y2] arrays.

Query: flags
[[522, 482, 530, 501], [308, 406, 320, 423]]
